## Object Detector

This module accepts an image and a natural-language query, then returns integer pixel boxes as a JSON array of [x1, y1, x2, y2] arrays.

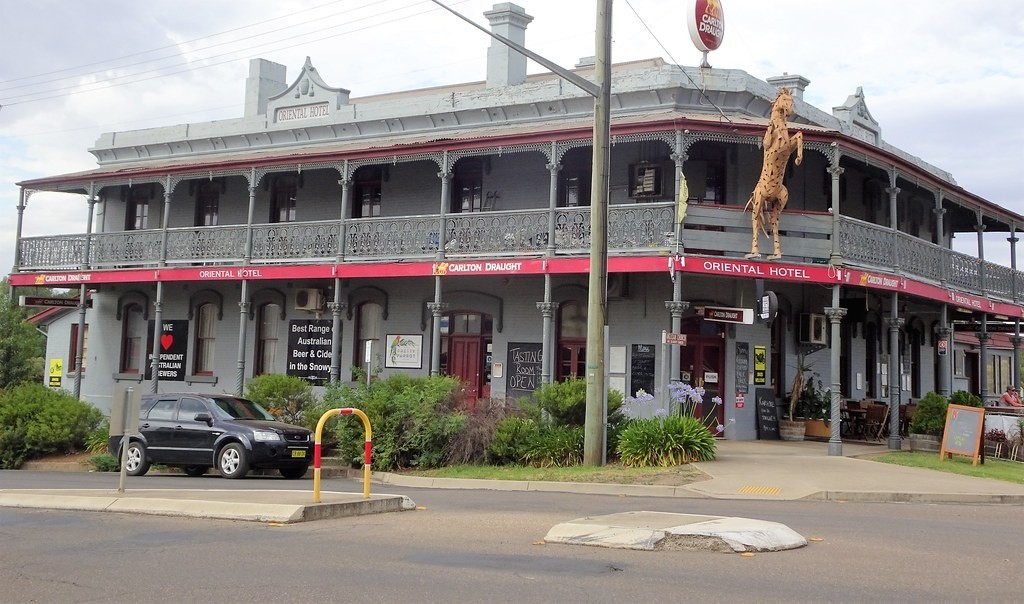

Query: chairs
[[840, 398, 918, 442]]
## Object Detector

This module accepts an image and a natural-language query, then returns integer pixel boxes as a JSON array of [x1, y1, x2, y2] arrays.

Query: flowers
[[621, 381, 736, 437], [985, 428, 1006, 442]]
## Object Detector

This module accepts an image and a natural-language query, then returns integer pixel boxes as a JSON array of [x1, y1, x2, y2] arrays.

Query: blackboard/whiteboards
[[286, 319, 343, 386], [144, 320, 189, 382], [942, 404, 984, 457], [505, 342, 543, 411], [755, 388, 781, 440], [735, 342, 749, 394], [631, 344, 655, 399]]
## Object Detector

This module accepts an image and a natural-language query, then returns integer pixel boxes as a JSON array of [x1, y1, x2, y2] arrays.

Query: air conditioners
[[608, 273, 632, 299], [294, 289, 326, 314], [800, 312, 828, 346]]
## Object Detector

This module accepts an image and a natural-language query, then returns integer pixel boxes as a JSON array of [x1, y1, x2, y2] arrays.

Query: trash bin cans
[[990, 400, 999, 415]]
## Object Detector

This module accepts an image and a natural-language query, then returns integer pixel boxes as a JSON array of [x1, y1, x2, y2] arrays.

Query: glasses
[[1011, 389, 1016, 392]]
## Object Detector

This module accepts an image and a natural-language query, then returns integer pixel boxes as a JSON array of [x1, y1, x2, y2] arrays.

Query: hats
[[1006, 385, 1015, 392]]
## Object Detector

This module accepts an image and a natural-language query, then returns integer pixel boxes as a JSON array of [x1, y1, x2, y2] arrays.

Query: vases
[[984, 439, 1009, 458]]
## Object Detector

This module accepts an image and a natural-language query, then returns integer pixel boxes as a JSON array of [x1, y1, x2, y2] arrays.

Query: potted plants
[[1006, 414, 1024, 462], [778, 351, 817, 441], [780, 372, 843, 439], [907, 390, 951, 453]]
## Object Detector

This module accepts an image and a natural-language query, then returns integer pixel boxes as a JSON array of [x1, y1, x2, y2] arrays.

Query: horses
[[741, 87, 803, 258]]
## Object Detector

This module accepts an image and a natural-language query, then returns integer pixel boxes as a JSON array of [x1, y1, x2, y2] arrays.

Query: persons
[[998, 385, 1024, 416]]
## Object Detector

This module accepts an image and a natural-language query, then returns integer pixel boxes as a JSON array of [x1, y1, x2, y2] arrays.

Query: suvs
[[110, 392, 315, 478]]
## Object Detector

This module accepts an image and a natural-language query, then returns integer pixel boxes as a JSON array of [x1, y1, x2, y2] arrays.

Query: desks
[[840, 409, 891, 442]]
